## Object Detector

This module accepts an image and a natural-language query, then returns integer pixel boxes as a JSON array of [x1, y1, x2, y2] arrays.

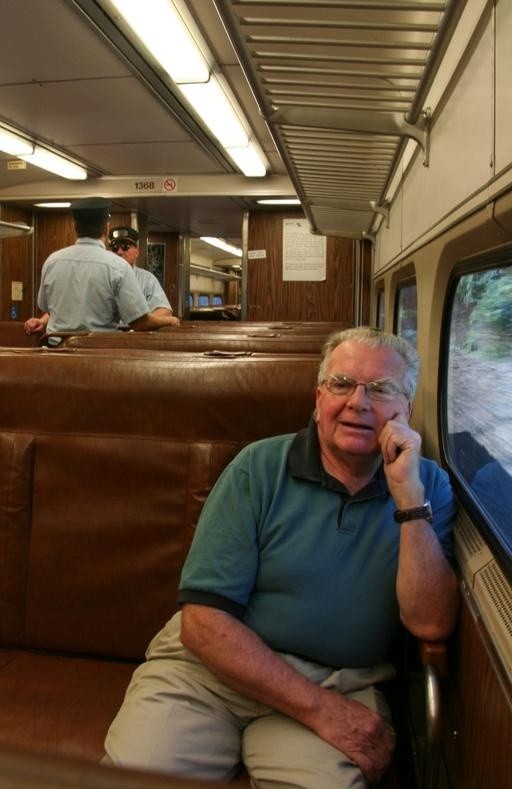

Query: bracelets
[[391, 499, 435, 527]]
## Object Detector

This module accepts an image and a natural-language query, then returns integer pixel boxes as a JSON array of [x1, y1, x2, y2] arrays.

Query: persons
[[21, 224, 175, 338], [95, 324, 461, 789], [35, 192, 181, 347]]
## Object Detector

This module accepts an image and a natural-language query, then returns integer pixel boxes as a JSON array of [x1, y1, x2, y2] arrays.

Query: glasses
[[316, 373, 410, 402], [110, 242, 131, 253]]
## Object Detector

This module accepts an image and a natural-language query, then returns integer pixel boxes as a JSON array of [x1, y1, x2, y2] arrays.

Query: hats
[[108, 225, 140, 248], [69, 197, 113, 216]]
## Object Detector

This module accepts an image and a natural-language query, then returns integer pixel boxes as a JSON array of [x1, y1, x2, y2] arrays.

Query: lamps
[[95, 2, 271, 181], [0, 118, 90, 186]]
